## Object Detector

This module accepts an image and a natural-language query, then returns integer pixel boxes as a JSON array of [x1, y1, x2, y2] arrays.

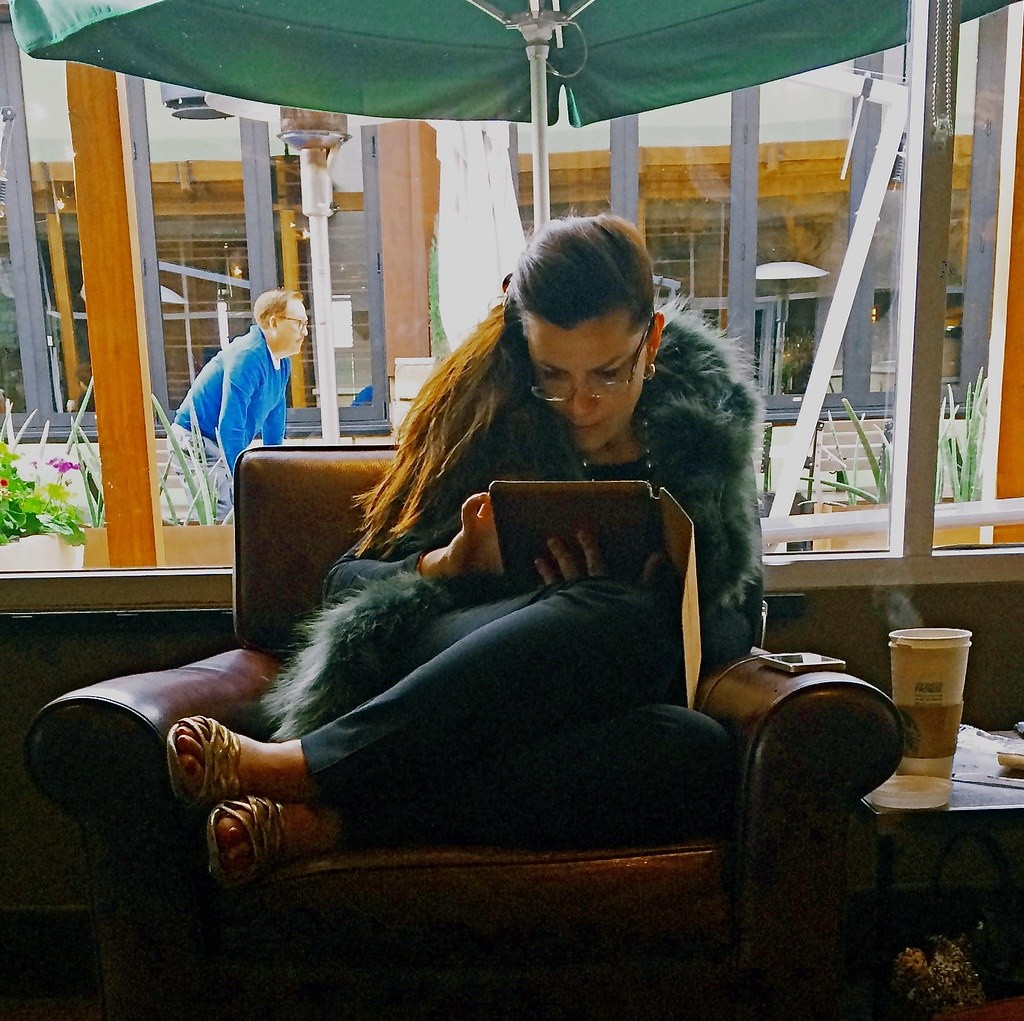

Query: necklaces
[[582, 406, 654, 483]]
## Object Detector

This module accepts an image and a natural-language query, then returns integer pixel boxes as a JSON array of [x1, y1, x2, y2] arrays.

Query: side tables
[[840, 726, 1024, 1021]]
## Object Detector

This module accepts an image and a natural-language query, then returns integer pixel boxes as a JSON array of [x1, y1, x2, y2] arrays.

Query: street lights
[[201, 90, 401, 446], [754, 261, 831, 395]]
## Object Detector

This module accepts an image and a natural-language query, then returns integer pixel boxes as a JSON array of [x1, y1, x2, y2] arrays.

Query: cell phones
[[758, 653, 846, 673]]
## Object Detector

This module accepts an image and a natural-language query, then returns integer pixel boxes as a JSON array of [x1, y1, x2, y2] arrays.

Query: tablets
[[490, 480, 656, 581]]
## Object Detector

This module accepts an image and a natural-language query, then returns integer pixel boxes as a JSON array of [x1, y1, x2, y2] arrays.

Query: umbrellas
[[9, 0, 1022, 233]]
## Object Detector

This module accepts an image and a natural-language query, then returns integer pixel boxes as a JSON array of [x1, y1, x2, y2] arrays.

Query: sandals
[[207, 794, 287, 889], [168, 715, 246, 808]]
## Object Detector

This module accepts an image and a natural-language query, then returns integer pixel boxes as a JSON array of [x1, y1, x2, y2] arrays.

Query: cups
[[888, 628, 973, 779], [67, 400, 75, 411]]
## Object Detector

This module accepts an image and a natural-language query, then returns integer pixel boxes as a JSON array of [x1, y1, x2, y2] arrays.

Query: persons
[[166, 216, 762, 884], [167, 291, 307, 525]]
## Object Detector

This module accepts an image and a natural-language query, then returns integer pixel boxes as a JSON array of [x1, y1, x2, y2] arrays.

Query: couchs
[[24, 444, 904, 1021]]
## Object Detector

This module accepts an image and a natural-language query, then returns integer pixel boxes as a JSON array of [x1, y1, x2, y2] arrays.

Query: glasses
[[282, 317, 308, 329], [528, 311, 656, 401]]
